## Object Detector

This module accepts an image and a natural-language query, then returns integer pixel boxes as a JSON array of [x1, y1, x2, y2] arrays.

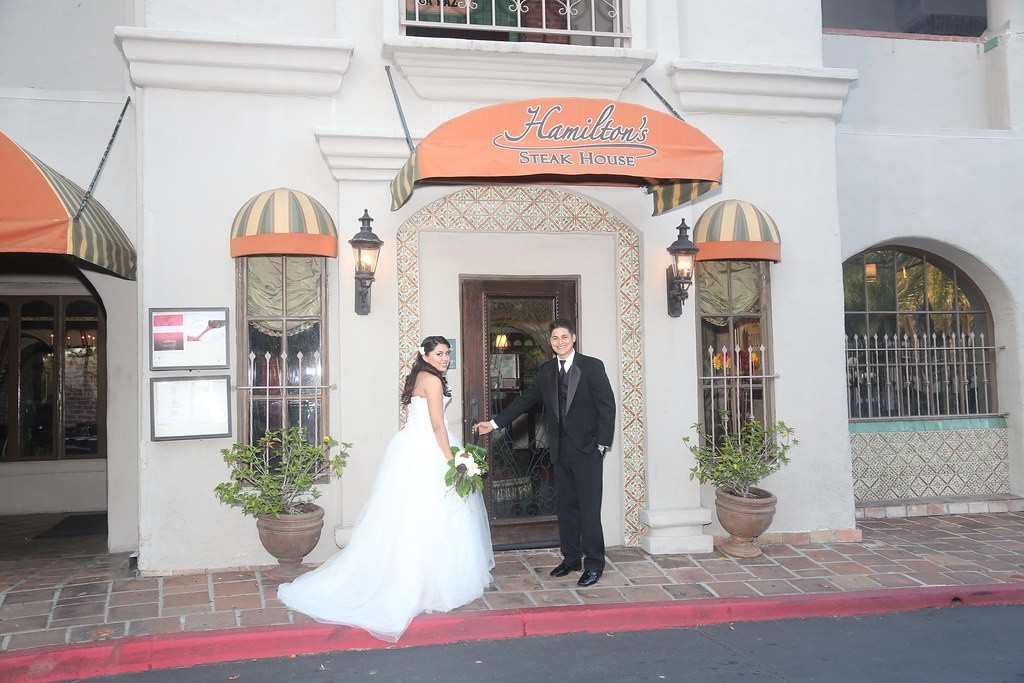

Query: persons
[[471, 320, 617, 587], [34, 394, 53, 451], [278, 336, 495, 644]]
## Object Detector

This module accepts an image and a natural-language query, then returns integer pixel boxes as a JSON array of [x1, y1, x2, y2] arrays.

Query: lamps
[[664, 218, 699, 319], [495, 328, 509, 350], [347, 209, 384, 315]]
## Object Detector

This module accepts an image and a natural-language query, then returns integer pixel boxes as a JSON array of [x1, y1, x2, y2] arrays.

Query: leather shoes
[[578, 569, 602, 587], [550, 564, 580, 577]]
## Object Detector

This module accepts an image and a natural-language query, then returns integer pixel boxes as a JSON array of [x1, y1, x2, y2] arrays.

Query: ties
[[558, 359, 566, 377]]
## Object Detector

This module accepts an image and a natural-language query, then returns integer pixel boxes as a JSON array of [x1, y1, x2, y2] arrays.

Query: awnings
[[390, 97, 724, 216], [693, 200, 782, 264], [230, 187, 338, 258], [0, 131, 136, 281]]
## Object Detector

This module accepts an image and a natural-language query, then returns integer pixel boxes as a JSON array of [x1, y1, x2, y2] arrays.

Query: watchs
[[597, 445, 606, 454]]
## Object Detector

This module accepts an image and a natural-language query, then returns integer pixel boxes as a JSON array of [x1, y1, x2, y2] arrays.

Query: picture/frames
[[149, 375, 233, 443], [149, 307, 231, 371]]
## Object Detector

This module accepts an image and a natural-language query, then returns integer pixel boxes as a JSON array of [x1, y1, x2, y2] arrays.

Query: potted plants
[[213, 425, 354, 583], [681, 409, 801, 559]]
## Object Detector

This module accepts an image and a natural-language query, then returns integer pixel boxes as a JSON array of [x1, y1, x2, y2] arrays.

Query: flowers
[[444, 443, 489, 498], [419, 346, 426, 356]]
